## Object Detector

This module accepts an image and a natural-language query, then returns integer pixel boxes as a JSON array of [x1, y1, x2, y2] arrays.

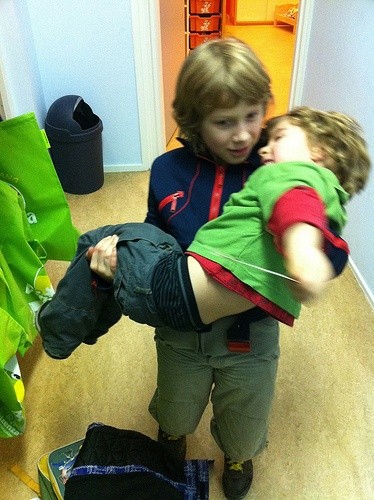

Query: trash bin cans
[[43, 94, 104, 195]]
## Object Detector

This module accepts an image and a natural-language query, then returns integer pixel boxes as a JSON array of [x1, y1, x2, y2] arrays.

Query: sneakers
[[222, 454, 254, 500], [158, 424, 186, 460]]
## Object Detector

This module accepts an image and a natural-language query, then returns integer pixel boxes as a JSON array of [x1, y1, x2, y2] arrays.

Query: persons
[[144, 38, 289, 500], [30, 107, 374, 360]]
